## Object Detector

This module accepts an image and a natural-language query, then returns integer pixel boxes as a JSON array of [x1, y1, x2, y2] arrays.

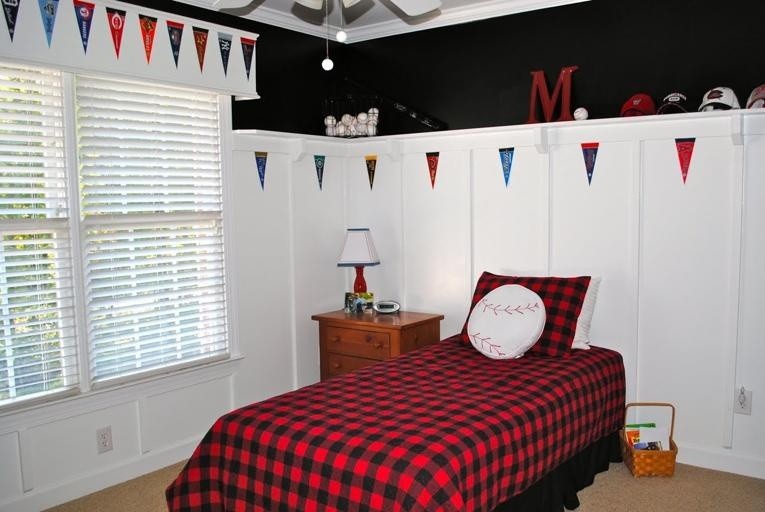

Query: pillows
[[466, 284, 547, 362], [571, 277, 601, 351], [461, 270, 591, 360]]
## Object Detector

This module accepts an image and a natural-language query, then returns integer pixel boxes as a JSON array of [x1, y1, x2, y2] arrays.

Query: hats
[[697, 87, 741, 111], [656, 92, 689, 113], [746, 85, 765, 109], [620, 93, 655, 116]]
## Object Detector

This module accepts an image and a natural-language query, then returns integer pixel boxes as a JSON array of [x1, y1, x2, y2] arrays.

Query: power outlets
[[95, 425, 113, 454]]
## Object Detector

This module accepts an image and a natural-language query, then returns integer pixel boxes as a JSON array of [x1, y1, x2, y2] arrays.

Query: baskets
[[618, 403, 677, 480], [323, 97, 381, 138]]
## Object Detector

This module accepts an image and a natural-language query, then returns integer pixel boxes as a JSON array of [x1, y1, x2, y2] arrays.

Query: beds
[[164, 333, 627, 512]]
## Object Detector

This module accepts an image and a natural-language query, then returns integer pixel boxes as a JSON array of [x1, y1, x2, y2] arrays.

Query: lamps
[[337, 228, 381, 293], [317, 0, 348, 72]]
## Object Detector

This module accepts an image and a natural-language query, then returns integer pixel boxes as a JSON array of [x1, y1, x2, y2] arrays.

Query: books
[[625, 422, 670, 451]]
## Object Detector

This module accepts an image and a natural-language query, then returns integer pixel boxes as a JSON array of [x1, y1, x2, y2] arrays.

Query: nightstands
[[310, 308, 445, 383]]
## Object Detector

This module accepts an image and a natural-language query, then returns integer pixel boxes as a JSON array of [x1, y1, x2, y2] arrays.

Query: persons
[[352, 293, 365, 312]]
